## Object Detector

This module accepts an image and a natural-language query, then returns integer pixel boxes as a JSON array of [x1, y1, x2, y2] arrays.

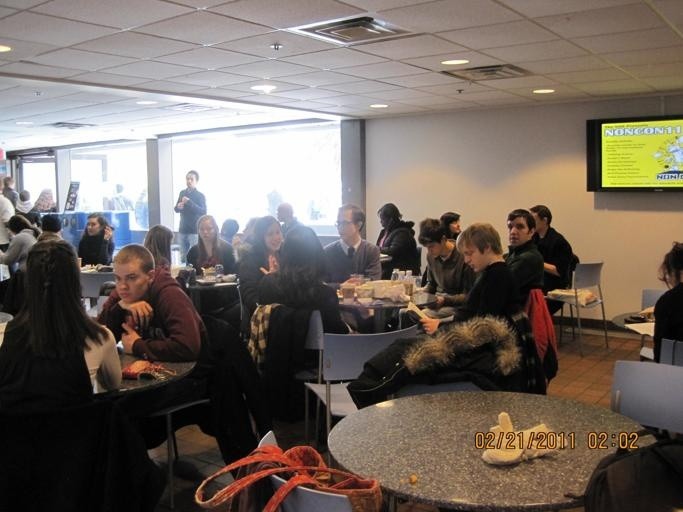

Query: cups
[[77, 257, 82, 268], [342, 287, 353, 303], [351, 274, 371, 287]]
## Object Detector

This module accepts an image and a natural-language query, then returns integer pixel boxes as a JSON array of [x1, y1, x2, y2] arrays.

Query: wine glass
[[215, 265, 224, 278]]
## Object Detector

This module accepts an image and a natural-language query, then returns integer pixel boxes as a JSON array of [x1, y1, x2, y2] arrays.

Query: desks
[[326, 390, 657, 512], [611, 310, 655, 339]]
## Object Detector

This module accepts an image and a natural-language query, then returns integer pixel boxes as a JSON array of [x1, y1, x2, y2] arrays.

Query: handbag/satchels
[[583, 429, 683, 512], [195, 445, 383, 512], [122, 360, 176, 382]]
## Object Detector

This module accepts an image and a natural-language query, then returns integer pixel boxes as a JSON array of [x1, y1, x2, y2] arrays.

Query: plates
[[196, 279, 215, 285]]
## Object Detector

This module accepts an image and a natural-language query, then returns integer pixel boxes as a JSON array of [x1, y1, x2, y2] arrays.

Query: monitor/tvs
[[586, 114, 683, 192]]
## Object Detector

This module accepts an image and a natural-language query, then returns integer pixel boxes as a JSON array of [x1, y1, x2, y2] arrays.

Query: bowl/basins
[[357, 298, 373, 307]]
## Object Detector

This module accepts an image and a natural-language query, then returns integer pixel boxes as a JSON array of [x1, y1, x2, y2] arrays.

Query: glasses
[[335, 221, 353, 226]]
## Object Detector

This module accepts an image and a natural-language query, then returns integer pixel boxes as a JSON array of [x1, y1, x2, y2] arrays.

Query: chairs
[[151, 316, 272, 510], [544, 260, 610, 358], [658, 338, 683, 368], [609, 357, 683, 436], [257, 430, 356, 512], [245, 303, 324, 446], [394, 316, 530, 397], [302, 323, 421, 448], [640, 288, 667, 359]]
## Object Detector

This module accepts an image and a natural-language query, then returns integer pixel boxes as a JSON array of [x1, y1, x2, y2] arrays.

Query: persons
[[109, 184, 136, 211], [653, 241, 683, 364], [145, 225, 188, 291], [174, 172, 207, 234], [0, 241, 122, 512], [377, 203, 417, 276], [78, 213, 114, 264], [99, 245, 213, 512], [322, 203, 381, 283], [417, 205, 574, 335], [260, 224, 359, 372], [1, 177, 65, 273], [185, 204, 301, 320]]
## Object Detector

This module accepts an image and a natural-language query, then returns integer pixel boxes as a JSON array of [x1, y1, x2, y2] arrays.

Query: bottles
[[391, 268, 413, 298]]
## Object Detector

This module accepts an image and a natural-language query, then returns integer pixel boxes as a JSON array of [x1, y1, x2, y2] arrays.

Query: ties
[[348, 246, 355, 259]]
